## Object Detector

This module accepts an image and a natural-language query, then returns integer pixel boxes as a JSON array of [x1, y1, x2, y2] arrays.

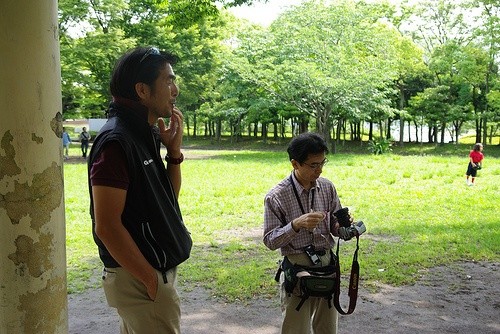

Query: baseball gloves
[[472, 163, 481, 170]]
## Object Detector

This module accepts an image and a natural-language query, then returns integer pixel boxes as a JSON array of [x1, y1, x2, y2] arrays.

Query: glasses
[[139, 47, 161, 63], [302, 157, 328, 168]]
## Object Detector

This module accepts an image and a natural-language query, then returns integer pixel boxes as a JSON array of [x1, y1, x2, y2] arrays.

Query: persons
[[84, 45, 193, 334], [78, 127, 92, 159], [61, 126, 73, 162], [465, 142, 484, 187], [260, 131, 365, 334]]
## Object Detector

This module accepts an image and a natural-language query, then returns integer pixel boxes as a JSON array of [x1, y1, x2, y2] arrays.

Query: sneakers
[[64, 155, 69, 159], [83, 153, 86, 157], [466, 181, 475, 187]]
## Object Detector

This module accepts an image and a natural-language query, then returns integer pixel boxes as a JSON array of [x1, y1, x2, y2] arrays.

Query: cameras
[[333, 207, 366, 241]]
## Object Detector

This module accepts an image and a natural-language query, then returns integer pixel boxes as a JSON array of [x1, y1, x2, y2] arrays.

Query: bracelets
[[164, 153, 185, 165]]
